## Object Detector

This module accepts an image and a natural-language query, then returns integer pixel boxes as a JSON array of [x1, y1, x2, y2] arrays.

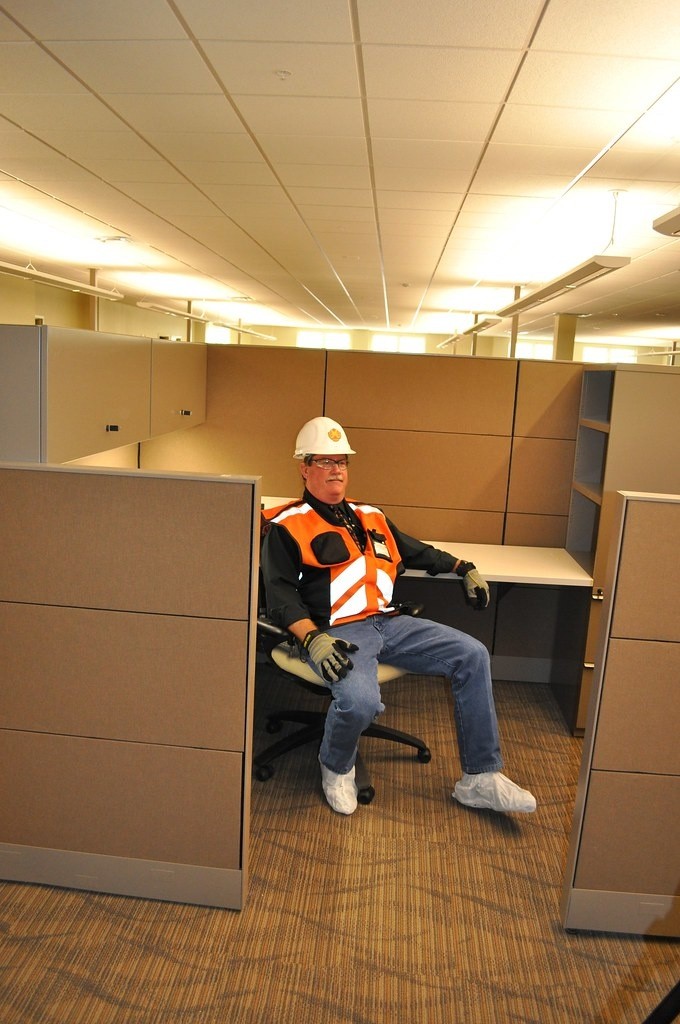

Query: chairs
[[253, 567, 431, 805]]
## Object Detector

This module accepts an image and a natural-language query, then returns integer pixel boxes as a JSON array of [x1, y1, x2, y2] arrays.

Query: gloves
[[458, 562, 490, 611], [302, 630, 359, 685]]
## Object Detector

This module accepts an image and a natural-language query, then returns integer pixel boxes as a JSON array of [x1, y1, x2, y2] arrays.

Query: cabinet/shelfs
[[1, 316, 207, 464], [549, 363, 680, 737]]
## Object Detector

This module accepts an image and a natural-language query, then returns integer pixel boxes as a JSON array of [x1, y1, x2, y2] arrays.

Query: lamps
[[435, 208, 680, 348], [0, 260, 277, 342]]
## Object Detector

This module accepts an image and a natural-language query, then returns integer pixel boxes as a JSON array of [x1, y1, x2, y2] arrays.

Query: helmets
[[292, 417, 356, 459]]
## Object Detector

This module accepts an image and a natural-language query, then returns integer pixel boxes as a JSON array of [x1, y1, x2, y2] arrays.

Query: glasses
[[310, 458, 352, 470]]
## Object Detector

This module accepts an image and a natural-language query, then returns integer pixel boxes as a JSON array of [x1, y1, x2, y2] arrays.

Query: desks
[[400, 539, 595, 587]]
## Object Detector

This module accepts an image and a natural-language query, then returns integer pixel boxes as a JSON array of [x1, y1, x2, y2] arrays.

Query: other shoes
[[452, 770, 537, 812], [317, 753, 358, 815]]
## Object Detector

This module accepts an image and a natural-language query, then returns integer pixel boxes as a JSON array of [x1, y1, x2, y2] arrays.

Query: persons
[[261, 417, 539, 815]]
